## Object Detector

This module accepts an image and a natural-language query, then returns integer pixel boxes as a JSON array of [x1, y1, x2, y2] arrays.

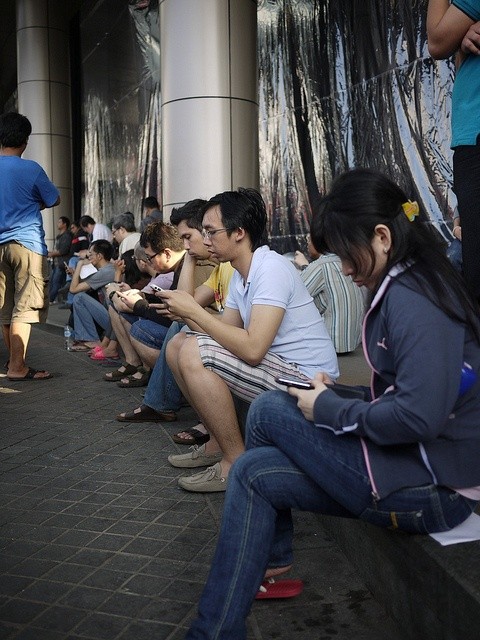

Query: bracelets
[[452, 226, 460, 237], [109, 291, 116, 303]]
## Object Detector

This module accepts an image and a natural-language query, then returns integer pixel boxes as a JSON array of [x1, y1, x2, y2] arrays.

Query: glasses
[[202, 229, 228, 237], [147, 253, 160, 263], [132, 255, 146, 263], [112, 226, 120, 235]]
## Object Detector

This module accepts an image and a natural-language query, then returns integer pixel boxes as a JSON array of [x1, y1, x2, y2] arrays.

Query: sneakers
[[177, 463, 227, 491], [168, 443, 224, 468]]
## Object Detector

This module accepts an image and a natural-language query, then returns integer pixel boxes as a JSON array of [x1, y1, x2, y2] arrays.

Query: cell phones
[[149, 284, 164, 293], [275, 377, 314, 389], [63, 260, 70, 270]]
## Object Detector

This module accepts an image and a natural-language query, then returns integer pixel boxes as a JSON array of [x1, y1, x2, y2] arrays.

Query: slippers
[[8, 367, 53, 381], [256, 578, 303, 597], [69, 341, 95, 352]]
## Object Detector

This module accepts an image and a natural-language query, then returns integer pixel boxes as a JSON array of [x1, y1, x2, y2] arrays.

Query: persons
[[87, 249, 152, 360], [292, 232, 371, 357], [0, 112, 62, 382], [58, 215, 115, 309], [103, 240, 175, 387], [110, 214, 142, 264], [69, 222, 89, 258], [184, 167, 479, 639], [139, 196, 163, 233], [425, 0, 479, 316], [445, 205, 463, 275], [116, 199, 238, 446], [67, 239, 123, 352], [47, 216, 74, 305], [153, 187, 341, 493], [114, 221, 190, 406]]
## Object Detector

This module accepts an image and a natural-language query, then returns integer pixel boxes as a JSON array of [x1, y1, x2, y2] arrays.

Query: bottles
[[64, 322, 74, 351]]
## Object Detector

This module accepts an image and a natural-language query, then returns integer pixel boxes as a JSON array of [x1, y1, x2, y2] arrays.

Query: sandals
[[172, 427, 210, 445], [91, 351, 119, 360], [116, 404, 177, 422], [87, 346, 103, 357], [103, 361, 143, 381], [116, 368, 153, 387]]
